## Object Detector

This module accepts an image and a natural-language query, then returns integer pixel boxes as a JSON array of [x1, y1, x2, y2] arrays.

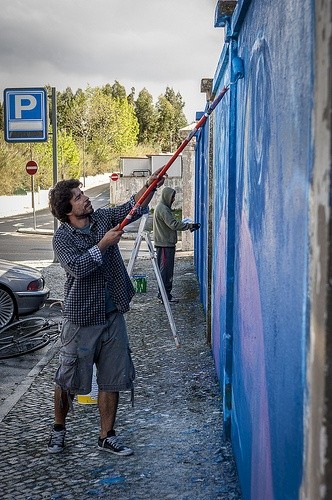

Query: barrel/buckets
[[133, 274, 147, 292], [78, 363, 99, 405]]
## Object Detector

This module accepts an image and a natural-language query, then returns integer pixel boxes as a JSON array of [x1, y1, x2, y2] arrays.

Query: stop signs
[[25, 160, 38, 175], [111, 173, 119, 182]]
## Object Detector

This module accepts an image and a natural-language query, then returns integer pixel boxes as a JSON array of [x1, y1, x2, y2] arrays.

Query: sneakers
[[157, 296, 180, 305], [97, 429, 134, 456], [46, 427, 66, 454]]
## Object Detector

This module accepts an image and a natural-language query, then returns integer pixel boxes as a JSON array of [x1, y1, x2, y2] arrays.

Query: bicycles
[[0, 301, 65, 360]]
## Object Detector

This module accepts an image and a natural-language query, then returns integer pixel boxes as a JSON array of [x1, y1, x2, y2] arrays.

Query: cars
[[0, 259, 52, 332]]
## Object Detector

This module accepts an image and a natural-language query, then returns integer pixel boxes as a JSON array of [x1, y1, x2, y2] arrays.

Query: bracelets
[[145, 180, 157, 191]]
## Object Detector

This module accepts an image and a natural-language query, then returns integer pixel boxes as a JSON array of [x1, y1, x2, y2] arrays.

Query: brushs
[[115, 56, 244, 234]]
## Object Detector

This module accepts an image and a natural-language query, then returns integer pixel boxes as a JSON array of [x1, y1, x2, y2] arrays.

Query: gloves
[[191, 223, 201, 230]]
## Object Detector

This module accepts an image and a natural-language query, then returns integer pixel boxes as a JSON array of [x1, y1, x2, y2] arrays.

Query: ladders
[[126, 215, 181, 349]]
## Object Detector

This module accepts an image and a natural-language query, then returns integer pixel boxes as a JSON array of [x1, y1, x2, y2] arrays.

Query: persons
[[154, 187, 200, 305], [47, 165, 168, 455]]
